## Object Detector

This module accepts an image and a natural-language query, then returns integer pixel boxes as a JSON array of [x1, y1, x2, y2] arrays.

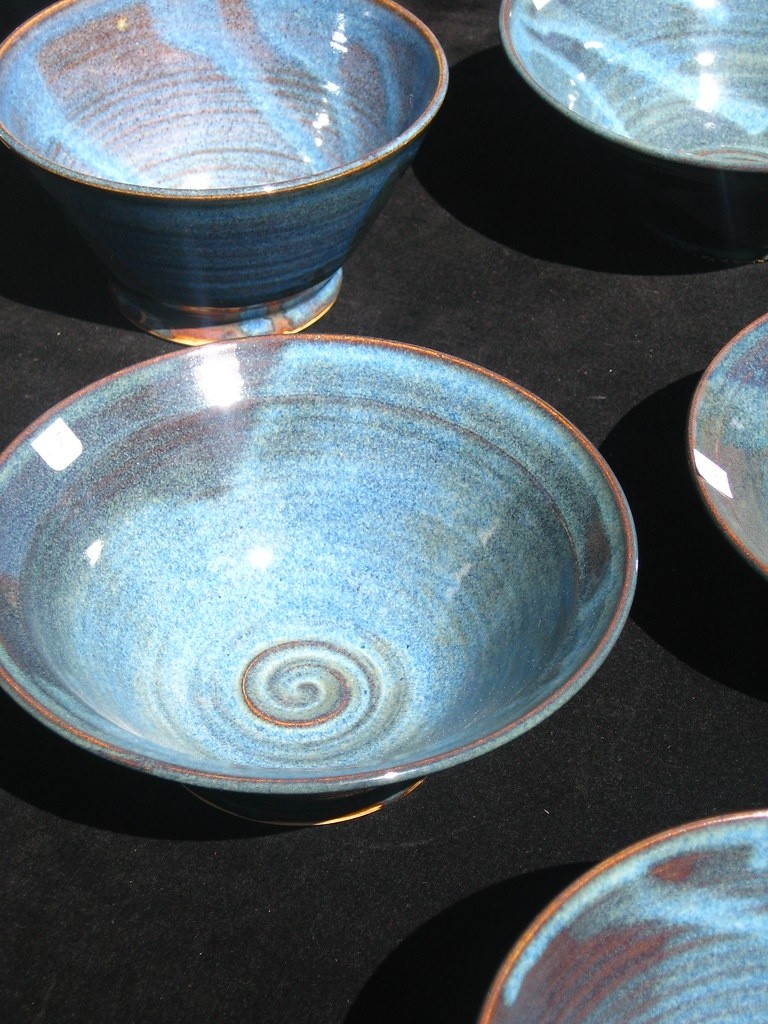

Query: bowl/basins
[[497, 0, 768, 172], [687, 312, 768, 580], [0, 0, 449, 346], [477, 808, 768, 1024], [0, 334, 641, 827]]
